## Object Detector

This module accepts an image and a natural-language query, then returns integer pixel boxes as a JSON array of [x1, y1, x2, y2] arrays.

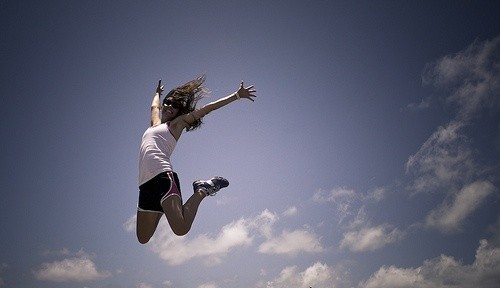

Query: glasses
[[163, 99, 179, 109]]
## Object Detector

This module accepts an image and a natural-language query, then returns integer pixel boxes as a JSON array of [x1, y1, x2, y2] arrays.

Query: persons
[[136, 74, 256, 244]]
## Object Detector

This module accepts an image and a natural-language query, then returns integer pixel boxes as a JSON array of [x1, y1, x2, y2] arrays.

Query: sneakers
[[193, 176, 229, 196]]
